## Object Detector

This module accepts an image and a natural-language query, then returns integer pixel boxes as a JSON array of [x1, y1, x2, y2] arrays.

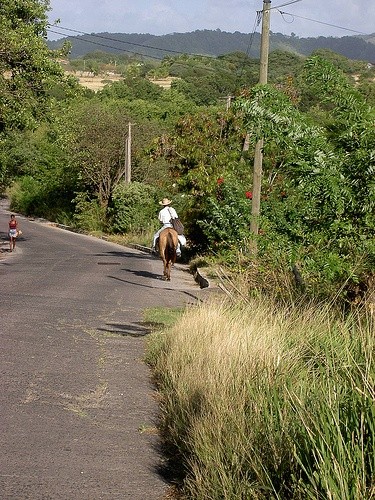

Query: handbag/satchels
[[169, 217, 184, 236]]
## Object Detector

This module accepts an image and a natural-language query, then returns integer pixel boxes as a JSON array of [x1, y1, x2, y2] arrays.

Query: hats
[[158, 197, 172, 205]]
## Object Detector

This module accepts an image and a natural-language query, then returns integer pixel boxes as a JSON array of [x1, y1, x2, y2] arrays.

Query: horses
[[157, 227, 179, 282]]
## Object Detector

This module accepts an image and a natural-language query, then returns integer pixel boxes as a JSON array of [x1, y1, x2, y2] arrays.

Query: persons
[[151, 197, 186, 257], [8, 214, 19, 252]]
